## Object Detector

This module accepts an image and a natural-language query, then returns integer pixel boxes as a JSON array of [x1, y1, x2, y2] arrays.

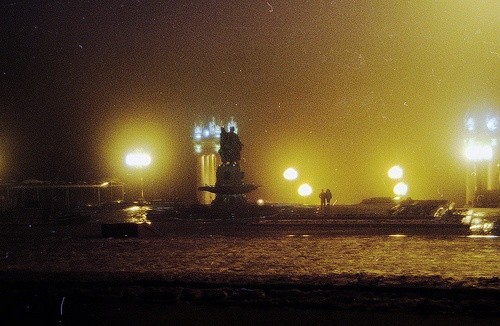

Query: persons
[[228, 126, 245, 164], [325, 189, 332, 205], [219, 127, 235, 166], [319, 190, 326, 208]]
